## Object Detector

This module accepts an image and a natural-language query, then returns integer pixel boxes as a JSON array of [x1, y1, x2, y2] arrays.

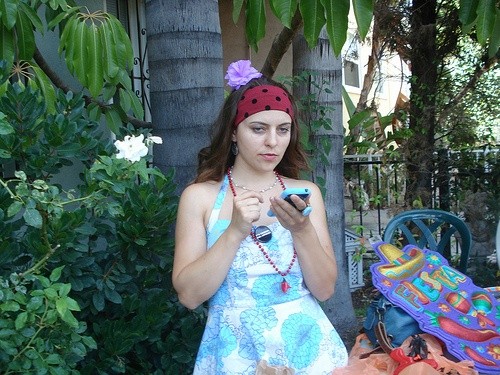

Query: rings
[[299, 204, 313, 216]]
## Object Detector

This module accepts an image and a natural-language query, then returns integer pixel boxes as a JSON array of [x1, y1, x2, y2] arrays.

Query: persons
[[172, 59, 351, 374]]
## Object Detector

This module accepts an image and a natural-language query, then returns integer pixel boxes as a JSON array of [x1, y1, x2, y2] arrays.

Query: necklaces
[[225, 165, 297, 294], [229, 165, 280, 192]]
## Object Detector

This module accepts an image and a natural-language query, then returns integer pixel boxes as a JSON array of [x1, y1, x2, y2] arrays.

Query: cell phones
[[268, 188, 311, 217]]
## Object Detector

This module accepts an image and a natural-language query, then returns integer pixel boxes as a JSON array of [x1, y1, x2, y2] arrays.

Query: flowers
[[223, 59, 262, 91]]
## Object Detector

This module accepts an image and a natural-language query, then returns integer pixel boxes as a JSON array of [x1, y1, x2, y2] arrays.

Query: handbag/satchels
[[362, 288, 424, 352]]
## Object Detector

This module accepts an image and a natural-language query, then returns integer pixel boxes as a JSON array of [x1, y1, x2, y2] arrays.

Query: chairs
[[382, 209, 472, 278]]
